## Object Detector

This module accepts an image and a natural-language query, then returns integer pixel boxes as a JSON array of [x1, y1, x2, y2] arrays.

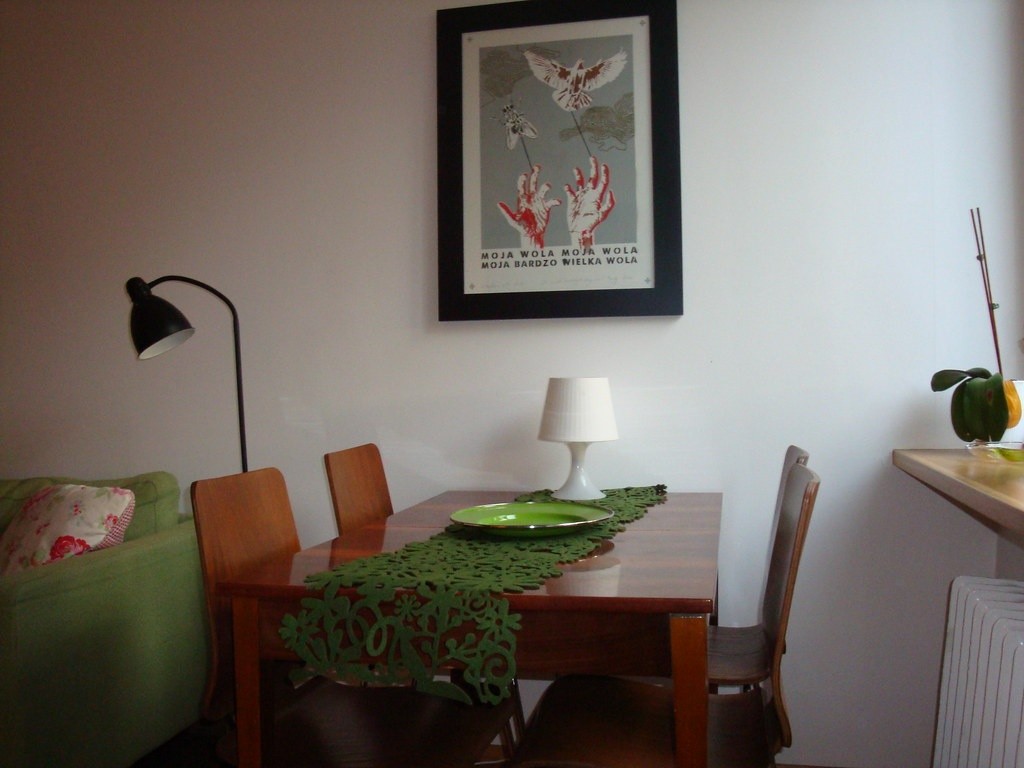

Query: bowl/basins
[[966, 442, 1024, 463]]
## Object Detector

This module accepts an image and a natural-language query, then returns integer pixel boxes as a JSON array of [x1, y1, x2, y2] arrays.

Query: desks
[[215, 490, 723, 768], [892, 449, 1024, 535]]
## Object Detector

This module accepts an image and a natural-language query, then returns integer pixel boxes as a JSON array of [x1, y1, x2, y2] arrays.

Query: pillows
[[0, 483, 135, 577]]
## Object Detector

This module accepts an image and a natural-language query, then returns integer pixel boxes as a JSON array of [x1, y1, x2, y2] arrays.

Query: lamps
[[537, 377, 619, 501], [125, 274, 248, 473]]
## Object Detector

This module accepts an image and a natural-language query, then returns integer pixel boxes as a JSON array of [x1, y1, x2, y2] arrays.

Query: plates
[[447, 501, 615, 537]]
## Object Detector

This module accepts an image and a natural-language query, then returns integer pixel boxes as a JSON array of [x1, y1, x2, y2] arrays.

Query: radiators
[[933, 574, 1024, 768]]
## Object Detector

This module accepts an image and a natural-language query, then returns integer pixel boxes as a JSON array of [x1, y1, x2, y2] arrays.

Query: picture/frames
[[434, 0, 682, 322]]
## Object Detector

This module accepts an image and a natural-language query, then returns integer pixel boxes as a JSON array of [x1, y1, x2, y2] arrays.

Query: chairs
[[190, 467, 516, 768], [506, 462, 821, 768], [621, 445, 809, 695], [323, 442, 394, 538]]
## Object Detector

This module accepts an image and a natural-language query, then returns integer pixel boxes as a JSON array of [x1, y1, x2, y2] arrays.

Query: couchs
[[0, 513, 210, 768]]
